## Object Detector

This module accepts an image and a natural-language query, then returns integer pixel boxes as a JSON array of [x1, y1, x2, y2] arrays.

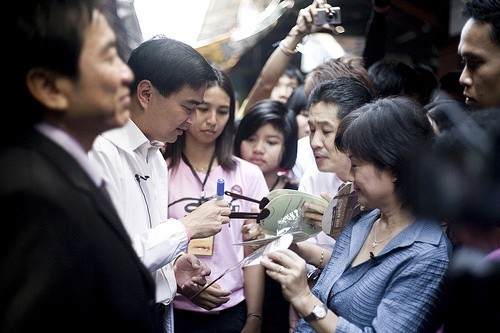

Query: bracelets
[[317, 247, 324, 269], [277, 41, 299, 56], [247, 313, 263, 321]]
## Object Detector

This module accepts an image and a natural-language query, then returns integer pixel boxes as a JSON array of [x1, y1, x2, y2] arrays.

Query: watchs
[[303, 302, 329, 323]]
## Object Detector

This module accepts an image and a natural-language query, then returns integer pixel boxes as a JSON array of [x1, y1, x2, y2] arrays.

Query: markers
[[217, 178, 225, 201]]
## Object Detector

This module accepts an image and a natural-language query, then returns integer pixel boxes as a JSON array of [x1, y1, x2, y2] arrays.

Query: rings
[[279, 266, 284, 273]]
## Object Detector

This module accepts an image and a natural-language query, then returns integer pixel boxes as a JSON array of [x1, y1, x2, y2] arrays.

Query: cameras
[[314, 6, 341, 26]]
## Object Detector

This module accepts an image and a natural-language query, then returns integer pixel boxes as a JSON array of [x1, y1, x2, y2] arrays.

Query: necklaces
[[195, 165, 208, 172], [270, 176, 281, 192], [372, 216, 406, 247]]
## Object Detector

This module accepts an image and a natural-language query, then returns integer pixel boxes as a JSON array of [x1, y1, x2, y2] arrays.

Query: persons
[[0, 0, 500, 333]]
